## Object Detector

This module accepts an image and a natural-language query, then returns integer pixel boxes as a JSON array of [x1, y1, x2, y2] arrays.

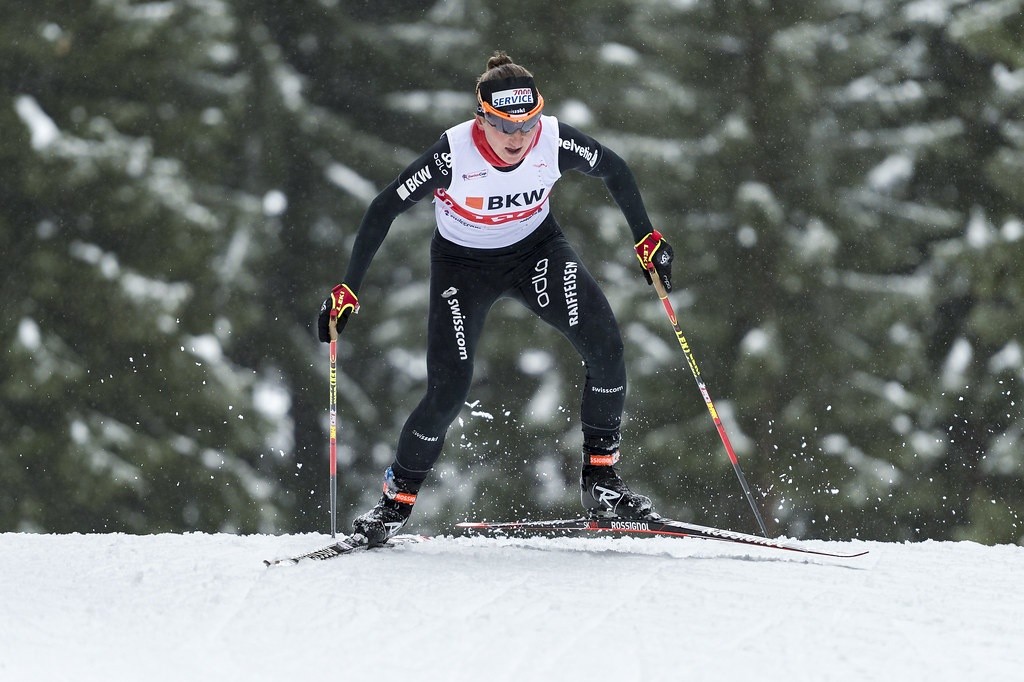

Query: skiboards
[[264, 517, 872, 565]]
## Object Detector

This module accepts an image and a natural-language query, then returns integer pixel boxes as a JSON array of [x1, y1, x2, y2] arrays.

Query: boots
[[580, 420, 655, 520], [352, 456, 432, 544]]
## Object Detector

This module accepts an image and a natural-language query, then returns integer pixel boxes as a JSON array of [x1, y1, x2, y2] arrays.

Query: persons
[[316, 50, 675, 540]]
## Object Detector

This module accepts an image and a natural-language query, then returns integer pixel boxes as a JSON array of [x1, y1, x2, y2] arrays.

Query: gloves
[[318, 283, 360, 344], [634, 229, 674, 294]]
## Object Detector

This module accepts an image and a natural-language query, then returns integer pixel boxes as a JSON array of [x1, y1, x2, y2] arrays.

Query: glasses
[[482, 94, 545, 134]]
